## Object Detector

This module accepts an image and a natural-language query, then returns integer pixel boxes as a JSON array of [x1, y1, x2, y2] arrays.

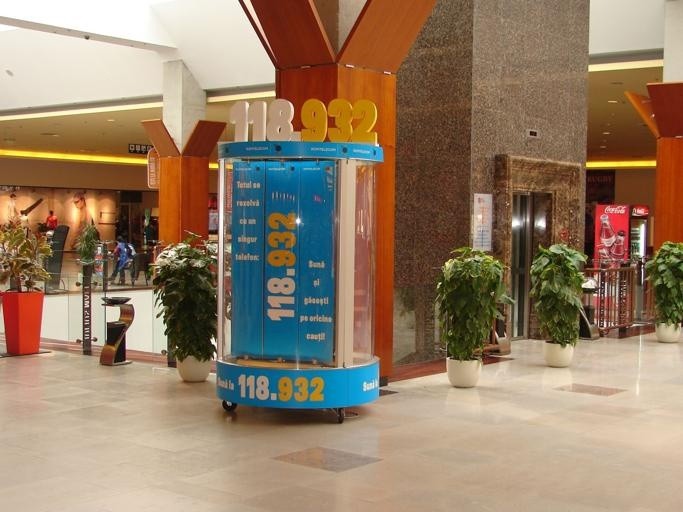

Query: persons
[[69, 192, 95, 249], [6, 194, 29, 240], [31, 210, 57, 240], [107, 214, 159, 286]]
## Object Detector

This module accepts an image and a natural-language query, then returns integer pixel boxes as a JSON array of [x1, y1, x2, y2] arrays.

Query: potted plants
[[433, 244, 517, 389], [0, 224, 53, 357], [643, 239, 682, 344], [527, 241, 589, 368], [148, 231, 217, 383]]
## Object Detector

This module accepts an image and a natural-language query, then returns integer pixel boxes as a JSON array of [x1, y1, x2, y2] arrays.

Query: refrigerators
[[593, 202, 650, 325]]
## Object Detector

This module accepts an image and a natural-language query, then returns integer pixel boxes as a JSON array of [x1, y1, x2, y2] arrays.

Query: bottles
[[598, 214, 626, 266]]
